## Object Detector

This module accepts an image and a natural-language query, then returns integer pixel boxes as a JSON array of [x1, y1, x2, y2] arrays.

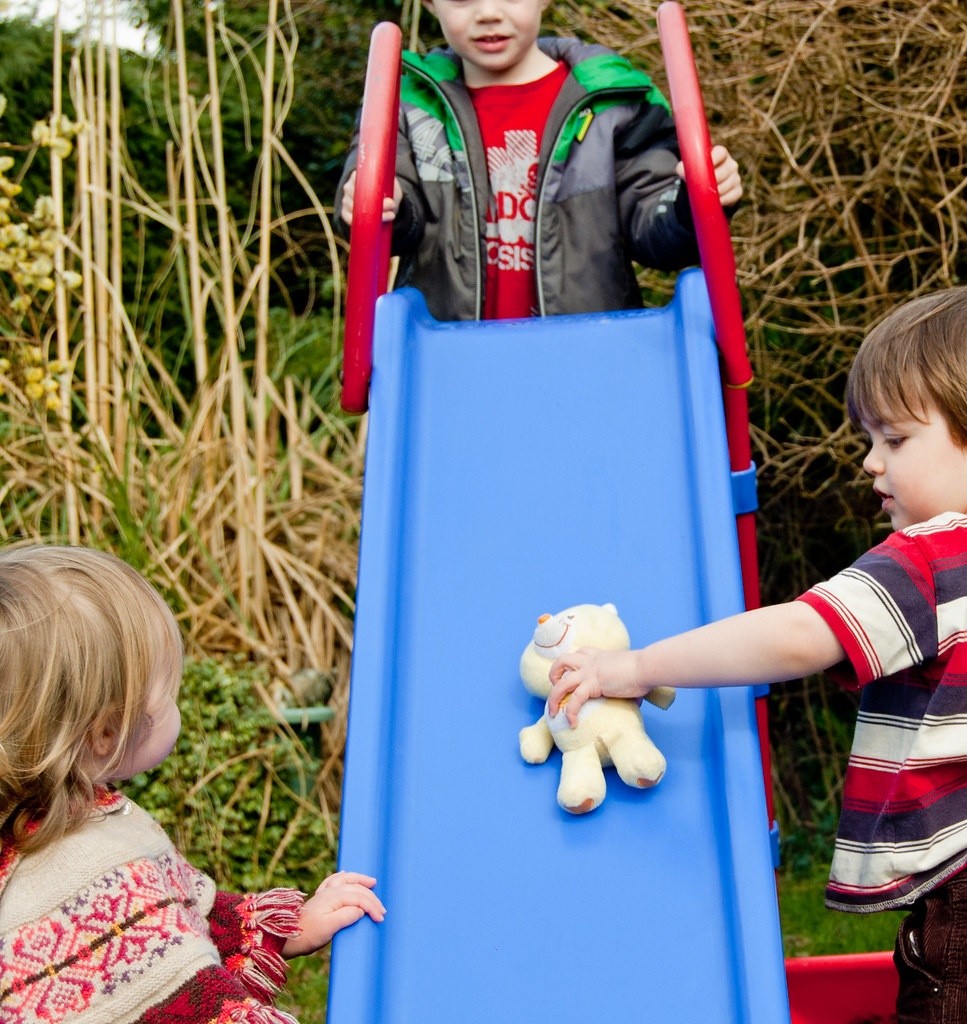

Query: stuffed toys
[[518, 602, 676, 815]]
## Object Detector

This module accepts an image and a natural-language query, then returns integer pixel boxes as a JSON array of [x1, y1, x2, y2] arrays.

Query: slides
[[325, 258, 794, 1024]]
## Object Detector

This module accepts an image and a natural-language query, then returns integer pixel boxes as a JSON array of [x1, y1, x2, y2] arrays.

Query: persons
[[546, 286, 967, 1023], [336, 1, 745, 324], [0, 548, 388, 1024]]
